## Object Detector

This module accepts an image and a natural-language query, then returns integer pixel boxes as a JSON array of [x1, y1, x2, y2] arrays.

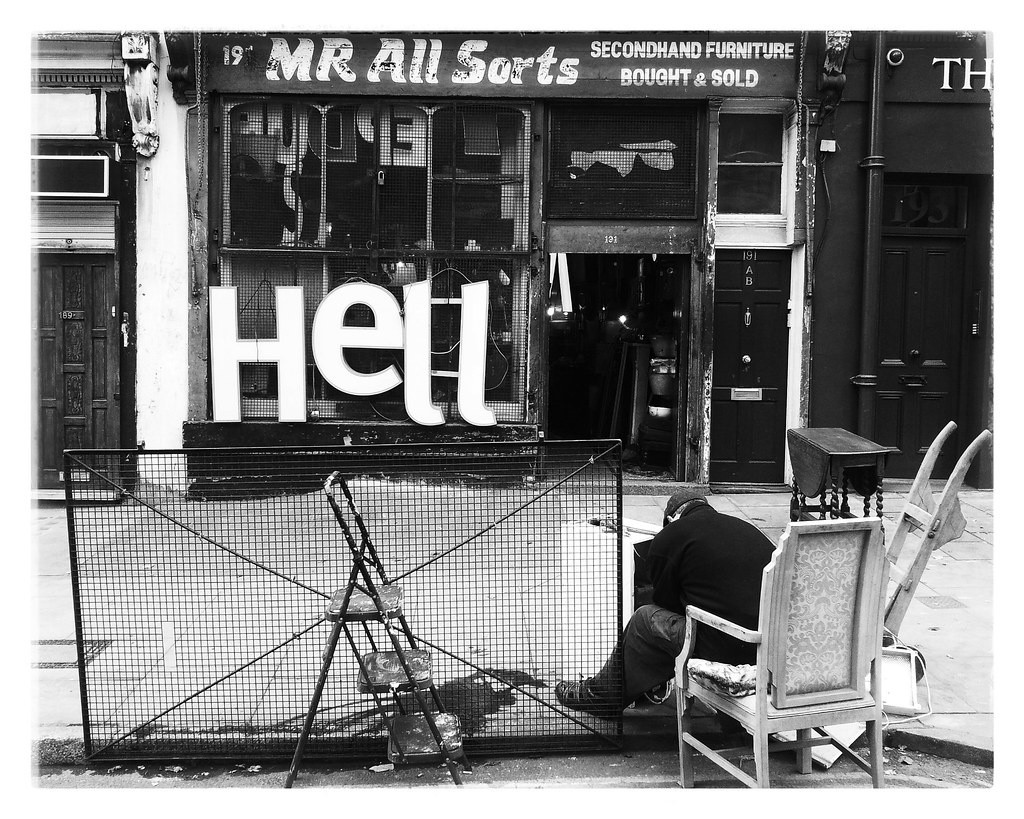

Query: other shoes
[[556, 677, 623, 722]]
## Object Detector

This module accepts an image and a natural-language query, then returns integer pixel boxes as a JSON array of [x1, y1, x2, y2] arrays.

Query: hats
[[663, 488, 708, 527]]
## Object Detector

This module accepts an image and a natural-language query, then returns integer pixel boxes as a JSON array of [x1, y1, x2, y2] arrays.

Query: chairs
[[676, 517, 886, 789]]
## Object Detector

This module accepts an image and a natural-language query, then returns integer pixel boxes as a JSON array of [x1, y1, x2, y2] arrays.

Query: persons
[[554, 491, 777, 720]]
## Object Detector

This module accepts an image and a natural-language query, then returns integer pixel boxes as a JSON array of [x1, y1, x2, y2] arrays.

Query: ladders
[[284, 470, 473, 789]]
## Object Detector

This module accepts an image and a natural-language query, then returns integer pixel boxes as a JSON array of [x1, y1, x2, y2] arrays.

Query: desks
[[786, 427, 903, 524]]
